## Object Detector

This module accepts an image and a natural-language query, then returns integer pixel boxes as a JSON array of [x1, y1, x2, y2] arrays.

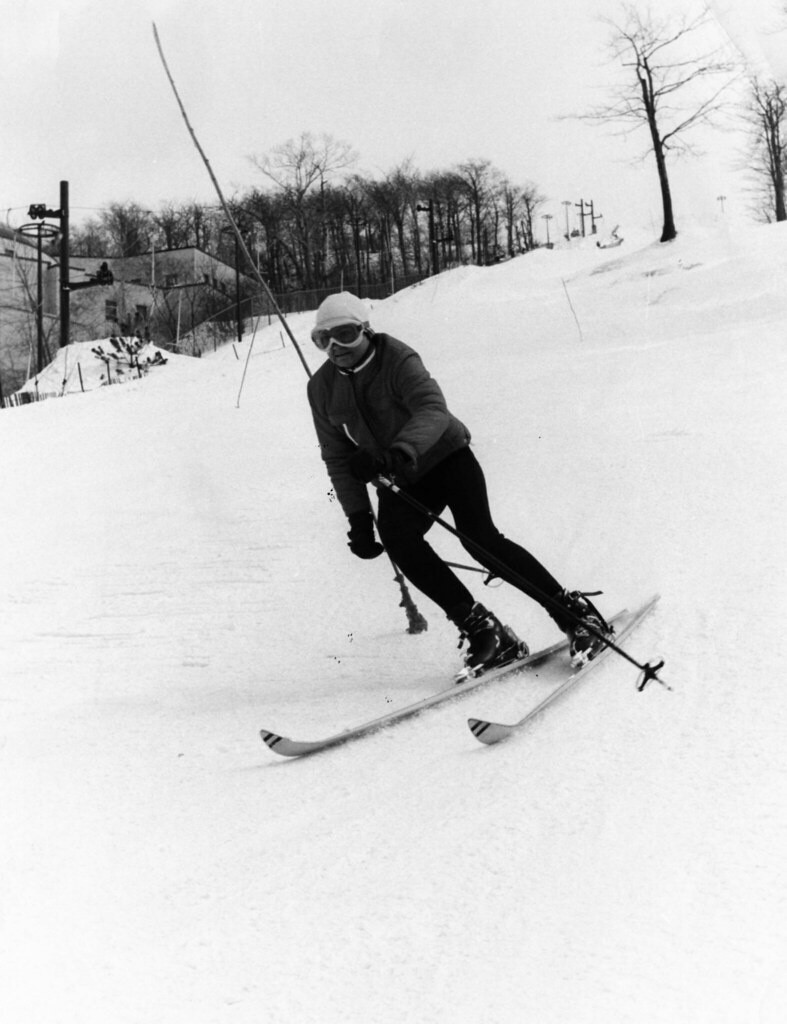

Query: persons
[[308, 292, 606, 681]]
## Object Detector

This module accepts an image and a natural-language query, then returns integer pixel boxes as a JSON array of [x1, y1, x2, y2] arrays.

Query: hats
[[316, 289, 370, 331]]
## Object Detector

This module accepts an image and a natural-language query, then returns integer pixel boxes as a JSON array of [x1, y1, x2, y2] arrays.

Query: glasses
[[310, 320, 370, 352]]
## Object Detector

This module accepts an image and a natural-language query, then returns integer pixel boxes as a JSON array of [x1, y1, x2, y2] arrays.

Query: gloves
[[347, 510, 383, 560], [377, 448, 412, 481]]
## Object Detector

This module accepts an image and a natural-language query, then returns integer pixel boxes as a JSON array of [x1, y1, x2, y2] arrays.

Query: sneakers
[[548, 587, 615, 666], [456, 602, 521, 673]]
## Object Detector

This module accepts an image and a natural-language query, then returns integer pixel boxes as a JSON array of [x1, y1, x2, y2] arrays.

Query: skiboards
[[256, 592, 665, 759]]
